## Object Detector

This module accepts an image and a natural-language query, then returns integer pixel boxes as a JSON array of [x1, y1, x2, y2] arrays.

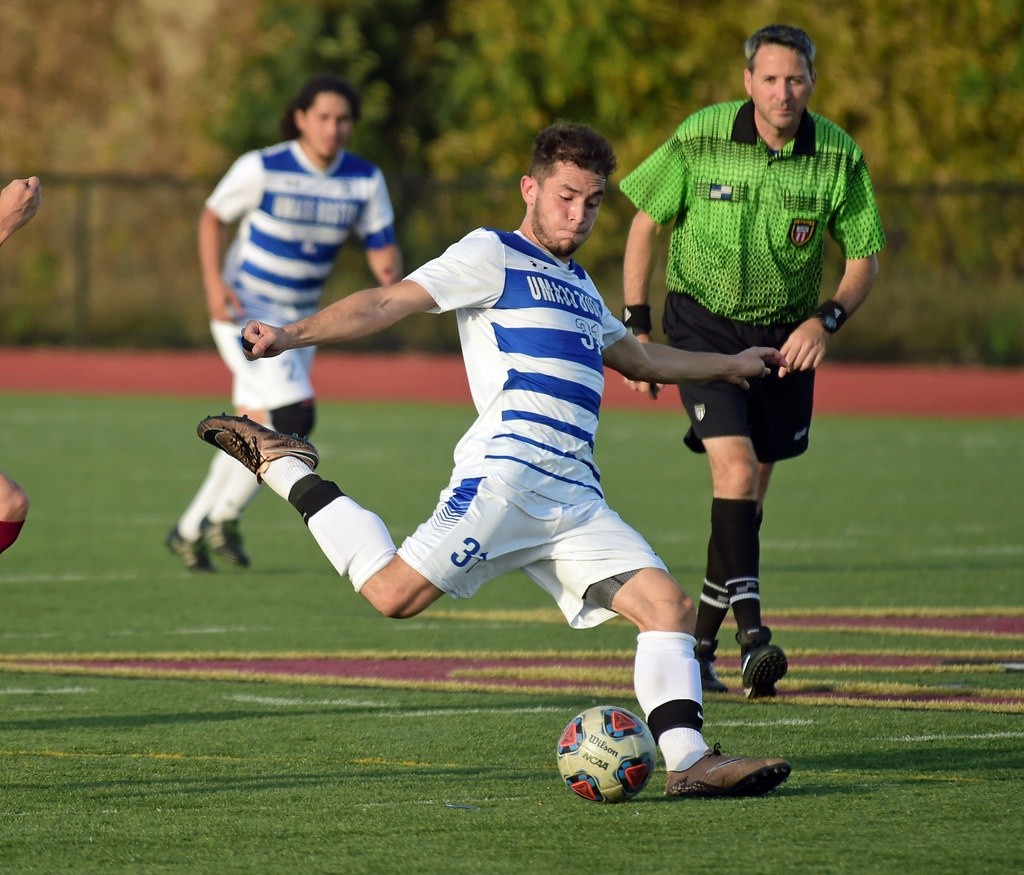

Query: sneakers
[[206, 519, 248, 566], [695, 638, 728, 691], [197, 412, 319, 484], [666, 743, 792, 799], [167, 529, 215, 573], [736, 626, 788, 698]]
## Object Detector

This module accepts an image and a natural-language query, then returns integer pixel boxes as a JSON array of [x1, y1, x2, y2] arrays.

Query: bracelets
[[817, 300, 848, 328], [622, 304, 652, 337]]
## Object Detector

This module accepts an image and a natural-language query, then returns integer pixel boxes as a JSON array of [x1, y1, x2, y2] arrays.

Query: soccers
[[556, 705, 658, 804]]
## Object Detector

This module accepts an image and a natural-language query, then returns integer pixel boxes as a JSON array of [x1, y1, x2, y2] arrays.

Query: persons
[[198, 122, 791, 798], [618, 25, 887, 700], [0, 176, 42, 556], [165, 77, 404, 573]]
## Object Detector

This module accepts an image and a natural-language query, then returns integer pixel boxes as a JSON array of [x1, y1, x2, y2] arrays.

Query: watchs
[[816, 313, 839, 334]]
